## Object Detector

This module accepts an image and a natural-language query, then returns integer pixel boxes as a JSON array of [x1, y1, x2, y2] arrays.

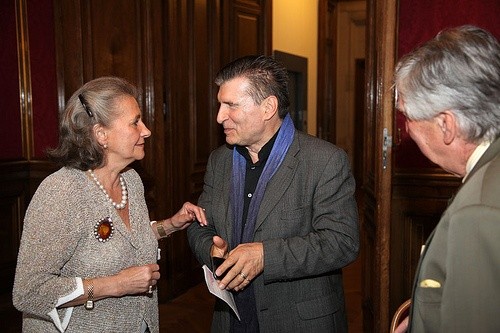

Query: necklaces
[[89, 169, 128, 210]]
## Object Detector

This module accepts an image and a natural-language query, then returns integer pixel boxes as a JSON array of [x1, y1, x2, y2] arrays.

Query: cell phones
[[211, 257, 236, 280]]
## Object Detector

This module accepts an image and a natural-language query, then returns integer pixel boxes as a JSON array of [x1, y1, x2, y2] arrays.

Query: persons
[[394, 25, 500, 333], [13, 77, 207, 333], [187, 56, 360, 333]]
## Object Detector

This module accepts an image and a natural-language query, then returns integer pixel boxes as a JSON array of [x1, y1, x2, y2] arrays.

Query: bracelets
[[157, 221, 171, 238]]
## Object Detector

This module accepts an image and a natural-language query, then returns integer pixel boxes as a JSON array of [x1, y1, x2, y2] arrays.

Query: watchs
[[85, 277, 95, 310]]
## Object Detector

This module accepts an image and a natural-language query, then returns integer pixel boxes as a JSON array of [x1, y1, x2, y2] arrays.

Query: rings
[[202, 208, 206, 211], [246, 278, 251, 283], [240, 272, 247, 279], [148, 286, 153, 294]]
